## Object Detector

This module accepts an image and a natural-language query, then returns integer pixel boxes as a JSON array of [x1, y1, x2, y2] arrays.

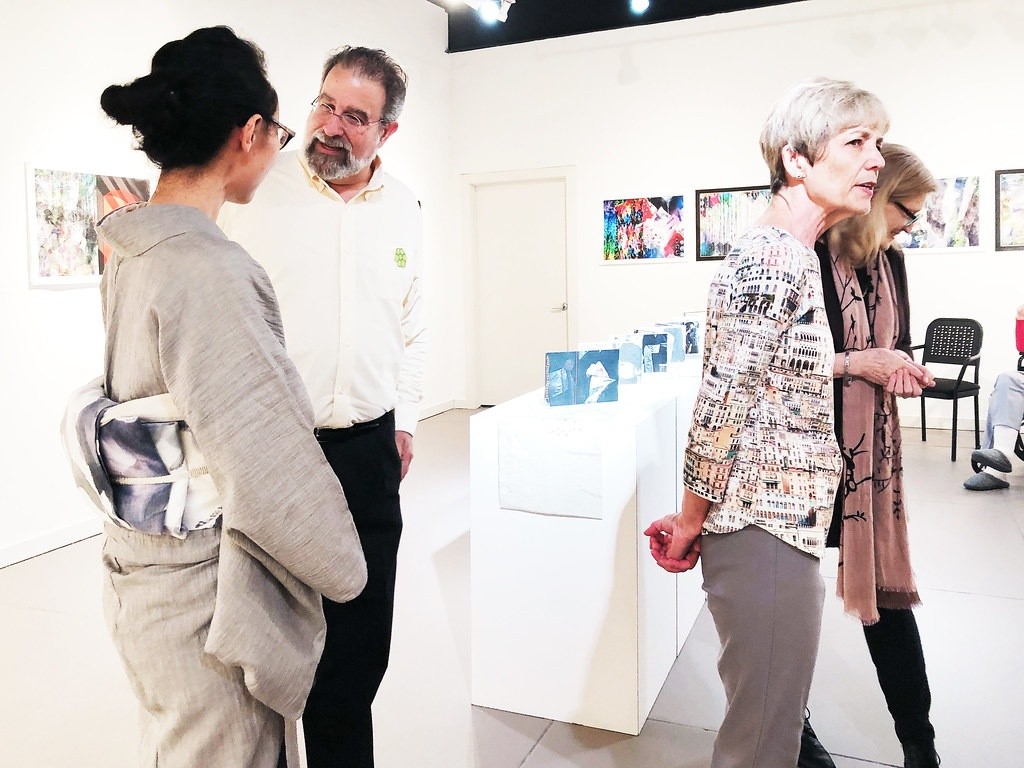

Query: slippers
[[963, 470, 1010, 491], [972, 448, 1012, 473]]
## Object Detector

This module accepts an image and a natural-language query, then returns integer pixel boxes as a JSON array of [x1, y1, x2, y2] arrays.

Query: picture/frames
[[695, 185, 771, 261], [596, 193, 691, 265], [24, 164, 155, 289], [995, 169, 1024, 251], [895, 175, 981, 253]]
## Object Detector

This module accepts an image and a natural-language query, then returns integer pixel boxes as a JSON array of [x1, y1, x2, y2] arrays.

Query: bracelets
[[844, 350, 852, 387]]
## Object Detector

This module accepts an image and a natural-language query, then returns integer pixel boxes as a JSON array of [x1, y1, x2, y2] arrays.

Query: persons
[[618, 342, 642, 384], [550, 357, 575, 407], [796, 143, 942, 768], [583, 361, 615, 404], [63, 24, 367, 768], [641, 75, 890, 768], [960, 306, 1024, 490], [685, 322, 699, 354], [215, 45, 430, 768]]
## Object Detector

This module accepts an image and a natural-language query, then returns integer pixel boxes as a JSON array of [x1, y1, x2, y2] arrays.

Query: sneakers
[[796, 709, 836, 768]]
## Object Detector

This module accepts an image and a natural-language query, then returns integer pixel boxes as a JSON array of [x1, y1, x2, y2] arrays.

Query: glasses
[[311, 94, 384, 135], [893, 199, 919, 227], [237, 114, 296, 151]]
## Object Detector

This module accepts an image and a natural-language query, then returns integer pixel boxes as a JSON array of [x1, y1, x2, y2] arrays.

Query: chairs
[[909, 317, 984, 462]]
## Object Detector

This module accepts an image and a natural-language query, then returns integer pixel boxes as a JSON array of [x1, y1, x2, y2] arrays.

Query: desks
[[467, 358, 706, 736]]
